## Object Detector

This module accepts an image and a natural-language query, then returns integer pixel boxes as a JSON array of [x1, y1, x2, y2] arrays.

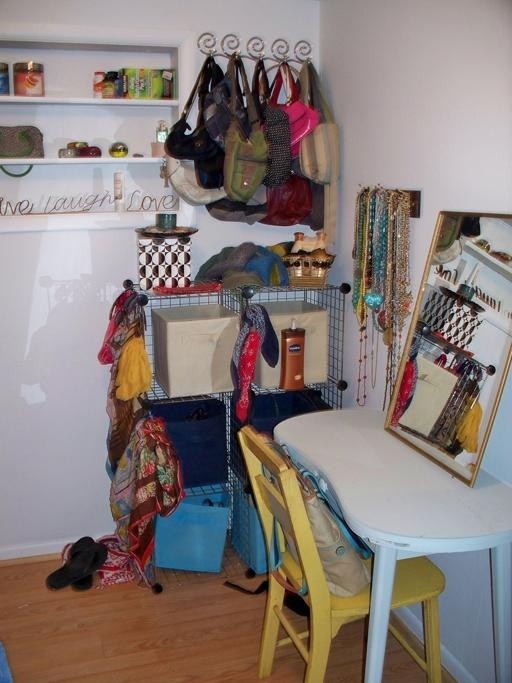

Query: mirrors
[[384, 211, 512, 488]]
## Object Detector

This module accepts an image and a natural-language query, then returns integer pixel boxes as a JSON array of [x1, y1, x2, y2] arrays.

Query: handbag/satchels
[[164, 78, 337, 232], [1, 125, 45, 158], [262, 440, 371, 597]]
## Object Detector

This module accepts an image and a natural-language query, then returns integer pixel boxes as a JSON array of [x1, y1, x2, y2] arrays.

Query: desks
[[273, 407, 512, 683]]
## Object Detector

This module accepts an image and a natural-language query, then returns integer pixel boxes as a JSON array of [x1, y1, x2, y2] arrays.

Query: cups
[[151, 142, 166, 157], [457, 284, 475, 299], [155, 214, 176, 229]]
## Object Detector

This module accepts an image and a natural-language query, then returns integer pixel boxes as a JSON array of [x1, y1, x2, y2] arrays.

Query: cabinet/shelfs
[[0, 22, 195, 234], [437, 235, 512, 338]]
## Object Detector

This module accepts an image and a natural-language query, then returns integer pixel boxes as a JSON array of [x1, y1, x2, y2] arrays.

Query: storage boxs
[[237, 493, 279, 576], [147, 390, 333, 489], [154, 492, 234, 573], [150, 304, 241, 398], [398, 355, 458, 437], [251, 300, 328, 389]]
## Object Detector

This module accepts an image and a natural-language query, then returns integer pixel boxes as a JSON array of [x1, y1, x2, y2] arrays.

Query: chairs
[[237, 425, 444, 683]]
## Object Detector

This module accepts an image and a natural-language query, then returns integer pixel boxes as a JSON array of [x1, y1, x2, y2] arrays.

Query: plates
[[135, 227, 199, 237], [439, 285, 486, 313]]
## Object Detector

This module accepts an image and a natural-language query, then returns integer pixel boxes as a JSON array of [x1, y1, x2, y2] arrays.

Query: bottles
[[314, 232, 327, 249], [467, 261, 485, 285], [114, 172, 125, 213], [93, 70, 124, 98], [291, 231, 307, 252]]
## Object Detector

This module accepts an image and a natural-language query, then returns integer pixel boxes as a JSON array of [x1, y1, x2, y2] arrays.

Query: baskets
[[282, 255, 333, 290]]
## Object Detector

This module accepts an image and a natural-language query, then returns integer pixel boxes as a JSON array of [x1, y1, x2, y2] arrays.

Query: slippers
[[46, 536, 108, 591]]
